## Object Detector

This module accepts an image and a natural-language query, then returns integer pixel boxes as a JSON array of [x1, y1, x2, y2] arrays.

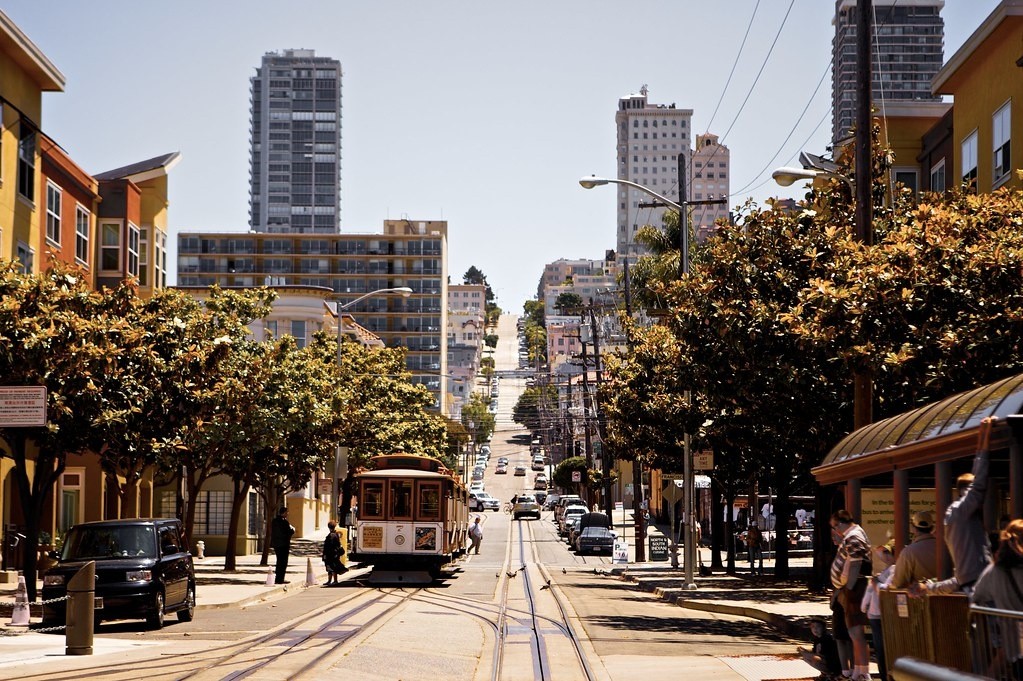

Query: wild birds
[[496, 573, 500, 577], [562, 567, 566, 574], [518, 565, 526, 571], [539, 580, 551, 590], [506, 571, 517, 579], [593, 568, 612, 577]]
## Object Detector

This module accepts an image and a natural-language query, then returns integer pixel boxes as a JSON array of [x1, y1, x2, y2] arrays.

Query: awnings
[[673, 475, 711, 488]]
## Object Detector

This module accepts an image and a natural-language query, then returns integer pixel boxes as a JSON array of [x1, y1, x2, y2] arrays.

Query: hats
[[912, 511, 933, 527], [751, 520, 757, 526], [877, 538, 896, 553]]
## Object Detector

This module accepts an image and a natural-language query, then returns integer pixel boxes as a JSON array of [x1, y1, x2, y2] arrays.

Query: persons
[[829, 509, 872, 681], [797, 615, 842, 681], [695, 515, 701, 547], [272, 507, 295, 584], [861, 538, 895, 681], [747, 521, 764, 577], [468, 517, 482, 555], [970, 519, 1023, 681], [322, 521, 340, 586], [892, 511, 954, 589], [510, 493, 526, 515], [926, 416, 999, 595]]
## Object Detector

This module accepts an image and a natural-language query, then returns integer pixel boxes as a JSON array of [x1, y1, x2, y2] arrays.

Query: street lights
[[771, 168, 855, 218], [580, 176, 696, 587], [331, 285, 413, 519]]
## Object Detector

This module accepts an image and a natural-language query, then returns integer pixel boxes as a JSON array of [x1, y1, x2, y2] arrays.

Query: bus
[[348, 449, 474, 578]]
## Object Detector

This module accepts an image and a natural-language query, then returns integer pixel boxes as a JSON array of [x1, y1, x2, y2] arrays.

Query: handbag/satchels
[[468, 529, 472, 539]]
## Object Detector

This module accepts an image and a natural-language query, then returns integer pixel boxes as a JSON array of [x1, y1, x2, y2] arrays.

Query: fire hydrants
[[668, 546, 679, 569]]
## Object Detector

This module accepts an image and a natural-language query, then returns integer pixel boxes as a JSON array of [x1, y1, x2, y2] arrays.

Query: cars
[[487, 313, 535, 423], [513, 466, 527, 476], [43, 517, 198, 630], [531, 438, 545, 470], [543, 492, 616, 557], [534, 473, 547, 503], [470, 445, 491, 492], [496, 457, 509, 474]]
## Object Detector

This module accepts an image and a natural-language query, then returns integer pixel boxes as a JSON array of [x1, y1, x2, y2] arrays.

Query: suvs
[[513, 496, 541, 519], [469, 492, 500, 512]]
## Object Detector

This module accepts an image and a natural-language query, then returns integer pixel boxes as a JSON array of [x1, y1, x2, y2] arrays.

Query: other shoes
[[323, 582, 330, 585], [813, 673, 832, 680], [468, 547, 470, 553], [832, 673, 871, 681], [279, 580, 290, 583], [331, 581, 338, 584], [751, 573, 755, 576], [758, 572, 763, 576], [475, 552, 480, 555]]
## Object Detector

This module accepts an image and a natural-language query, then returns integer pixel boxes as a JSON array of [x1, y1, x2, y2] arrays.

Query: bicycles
[[503, 502, 514, 515]]
